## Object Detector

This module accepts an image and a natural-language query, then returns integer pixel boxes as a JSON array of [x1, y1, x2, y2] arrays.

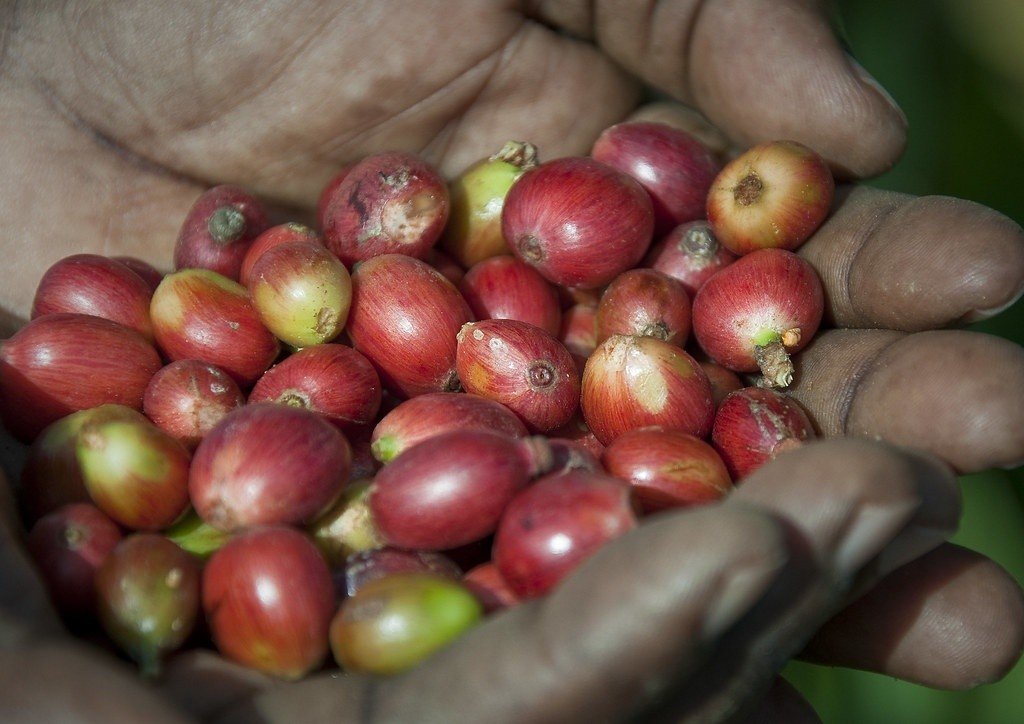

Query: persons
[[0, 0, 1023, 724]]
